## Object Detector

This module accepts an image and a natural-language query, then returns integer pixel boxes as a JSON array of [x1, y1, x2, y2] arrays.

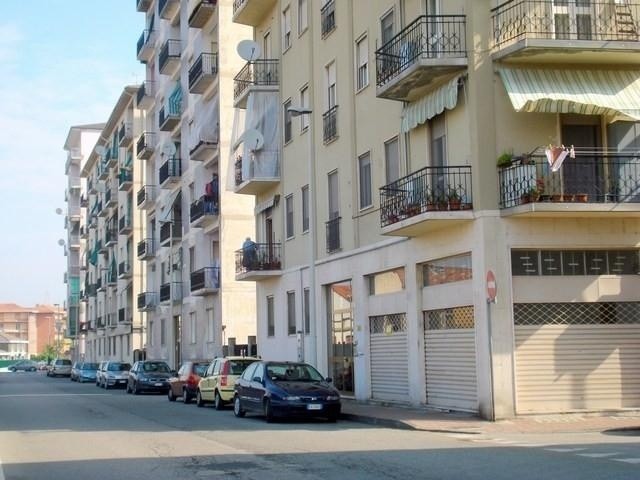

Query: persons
[[240, 236, 258, 263]]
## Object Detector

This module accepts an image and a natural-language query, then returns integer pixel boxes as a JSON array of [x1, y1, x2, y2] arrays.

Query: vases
[[540, 193, 588, 203]]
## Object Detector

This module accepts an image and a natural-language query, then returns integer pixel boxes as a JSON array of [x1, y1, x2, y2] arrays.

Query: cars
[[233, 360, 342, 423], [46, 358, 132, 389], [196, 355, 264, 410], [7, 359, 40, 372], [167, 358, 215, 404], [126, 360, 173, 395]]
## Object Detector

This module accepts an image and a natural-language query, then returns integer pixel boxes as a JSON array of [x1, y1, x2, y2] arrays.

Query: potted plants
[[425, 186, 462, 211], [521, 186, 540, 203]]
